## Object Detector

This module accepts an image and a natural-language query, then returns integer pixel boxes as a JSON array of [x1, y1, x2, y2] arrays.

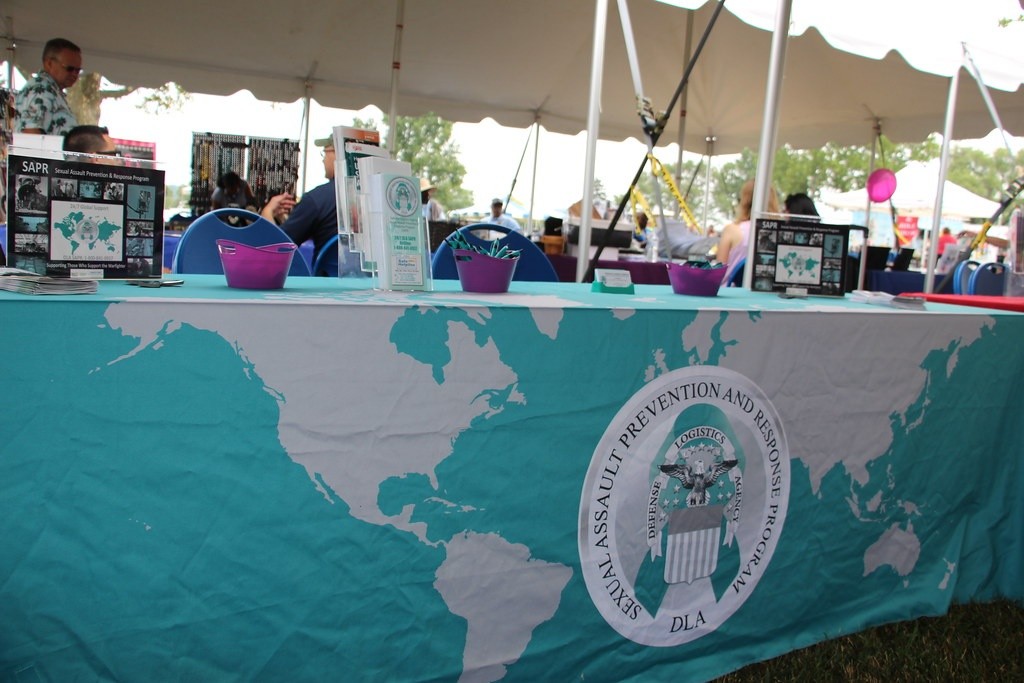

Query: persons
[[631, 212, 651, 249], [14, 37, 82, 137], [421, 179, 447, 220], [718, 180, 820, 288], [480, 198, 524, 241], [211, 172, 255, 228], [935, 227, 957, 267], [260, 133, 338, 270], [62, 126, 172, 275]]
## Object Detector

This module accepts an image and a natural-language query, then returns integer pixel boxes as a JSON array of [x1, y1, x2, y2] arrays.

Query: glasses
[[62, 66, 83, 73], [320, 148, 335, 158], [96, 151, 122, 158]]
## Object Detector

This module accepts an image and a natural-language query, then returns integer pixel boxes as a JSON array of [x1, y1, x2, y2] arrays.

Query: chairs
[[432, 222, 560, 282], [172, 206, 310, 275], [312, 231, 343, 277], [953, 257, 1019, 295]]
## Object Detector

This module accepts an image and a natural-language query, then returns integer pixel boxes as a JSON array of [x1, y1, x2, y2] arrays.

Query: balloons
[[866, 168, 897, 202]]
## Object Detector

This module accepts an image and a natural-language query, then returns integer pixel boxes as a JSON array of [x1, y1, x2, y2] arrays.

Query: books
[[332, 125, 429, 291]]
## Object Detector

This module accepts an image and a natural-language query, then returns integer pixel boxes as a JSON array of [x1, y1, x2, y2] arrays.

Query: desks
[[0, 275, 1024, 683]]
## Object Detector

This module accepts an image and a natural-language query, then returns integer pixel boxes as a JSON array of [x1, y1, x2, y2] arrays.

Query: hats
[[313, 134, 334, 146], [491, 198, 503, 208], [419, 178, 437, 193]]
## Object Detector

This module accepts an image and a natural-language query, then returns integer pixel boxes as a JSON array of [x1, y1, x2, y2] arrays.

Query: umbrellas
[[821, 159, 1002, 268]]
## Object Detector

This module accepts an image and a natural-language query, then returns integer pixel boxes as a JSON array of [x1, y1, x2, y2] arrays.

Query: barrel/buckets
[[215, 238, 298, 290], [452, 249, 520, 293], [542, 235, 564, 253], [664, 261, 730, 296]]
